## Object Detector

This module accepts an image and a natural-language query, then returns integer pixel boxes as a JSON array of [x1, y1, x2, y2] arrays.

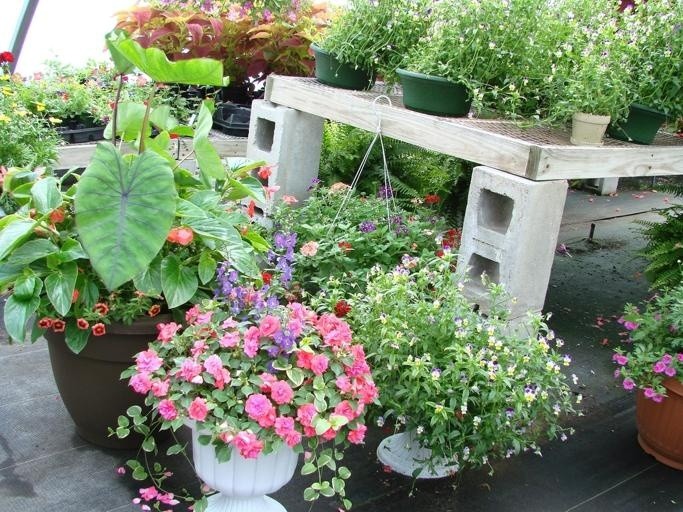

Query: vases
[[569, 111, 611, 142], [44, 116, 161, 142], [216, 81, 254, 103], [611, 98, 671, 145], [392, 65, 474, 116], [634, 378, 683, 471], [306, 40, 378, 93]]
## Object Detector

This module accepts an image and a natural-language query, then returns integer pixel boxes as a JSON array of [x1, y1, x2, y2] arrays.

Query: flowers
[[0, 50, 68, 200], [401, 0, 553, 117], [0, 26, 278, 357], [304, 0, 438, 92], [14, 56, 176, 124], [609, 0, 683, 124], [609, 275, 683, 404], [534, 0, 656, 143], [113, 0, 329, 78]]
